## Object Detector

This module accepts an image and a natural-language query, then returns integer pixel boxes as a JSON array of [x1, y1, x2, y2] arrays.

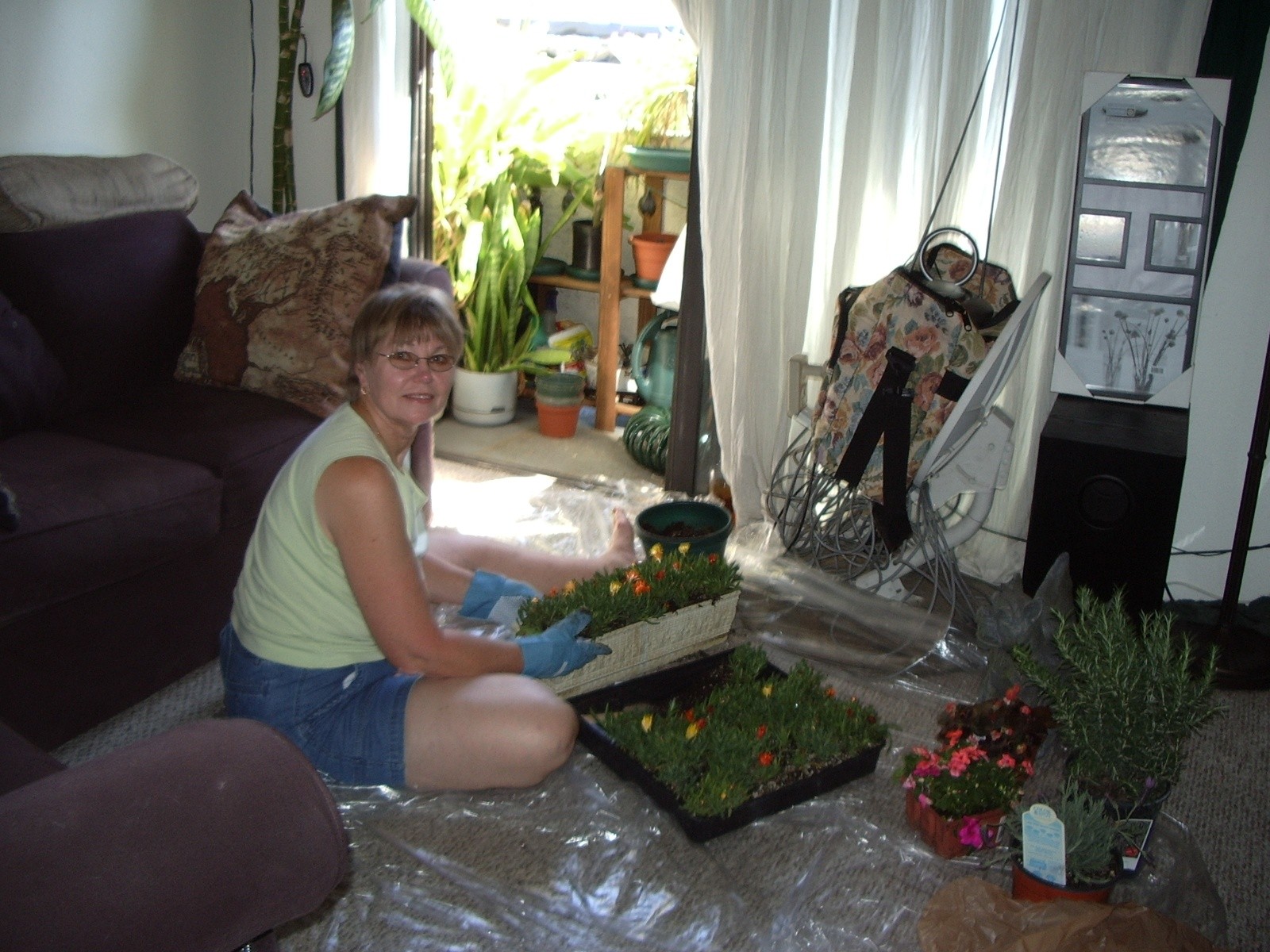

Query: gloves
[[516, 609, 612, 679], [458, 570, 543, 629]]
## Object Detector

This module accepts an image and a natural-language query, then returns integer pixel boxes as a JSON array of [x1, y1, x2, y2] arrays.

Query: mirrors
[[1051, 70, 1231, 407]]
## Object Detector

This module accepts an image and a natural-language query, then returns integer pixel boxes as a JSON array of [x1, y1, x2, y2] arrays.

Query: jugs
[[630, 309, 679, 409]]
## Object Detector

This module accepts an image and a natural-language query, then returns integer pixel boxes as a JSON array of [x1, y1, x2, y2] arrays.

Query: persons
[[216, 285, 638, 791]]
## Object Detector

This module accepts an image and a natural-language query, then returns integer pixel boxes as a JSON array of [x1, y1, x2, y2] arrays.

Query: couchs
[[0, 152, 443, 749], [0, 717, 349, 952]]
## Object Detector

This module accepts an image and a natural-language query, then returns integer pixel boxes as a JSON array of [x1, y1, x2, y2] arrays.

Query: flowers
[[895, 731, 1036, 819], [1102, 322, 1145, 386], [515, 543, 744, 641], [1114, 307, 1190, 369], [590, 642, 904, 822], [974, 775, 1158, 886], [937, 687, 1058, 772]]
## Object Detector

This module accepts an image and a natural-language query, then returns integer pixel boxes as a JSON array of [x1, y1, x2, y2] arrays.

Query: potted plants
[[1012, 584, 1244, 880], [448, 167, 574, 426], [604, 83, 695, 160]]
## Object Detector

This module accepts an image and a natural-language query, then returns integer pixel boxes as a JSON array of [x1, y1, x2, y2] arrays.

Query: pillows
[[171, 188, 418, 420], [0, 153, 199, 233]]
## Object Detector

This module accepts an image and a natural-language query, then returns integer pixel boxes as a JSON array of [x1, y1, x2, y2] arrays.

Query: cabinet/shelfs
[[515, 164, 690, 431]]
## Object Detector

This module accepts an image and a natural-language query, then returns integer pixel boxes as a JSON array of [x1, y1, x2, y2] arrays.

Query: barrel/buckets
[[634, 501, 733, 561]]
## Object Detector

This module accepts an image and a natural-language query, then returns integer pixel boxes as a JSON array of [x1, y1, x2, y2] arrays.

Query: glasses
[[376, 351, 458, 372]]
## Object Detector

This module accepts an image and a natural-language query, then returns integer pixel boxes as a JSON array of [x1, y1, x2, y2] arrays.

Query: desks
[[1022, 392, 1189, 639]]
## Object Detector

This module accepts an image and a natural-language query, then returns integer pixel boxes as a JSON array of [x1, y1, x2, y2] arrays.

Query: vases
[[1131, 364, 1159, 391], [900, 776, 1005, 859], [572, 220, 602, 273], [1101, 354, 1123, 388], [628, 233, 679, 282], [540, 586, 741, 700], [564, 645, 887, 844], [634, 501, 735, 562], [534, 373, 585, 438], [1011, 854, 1116, 905], [944, 699, 1043, 802]]
